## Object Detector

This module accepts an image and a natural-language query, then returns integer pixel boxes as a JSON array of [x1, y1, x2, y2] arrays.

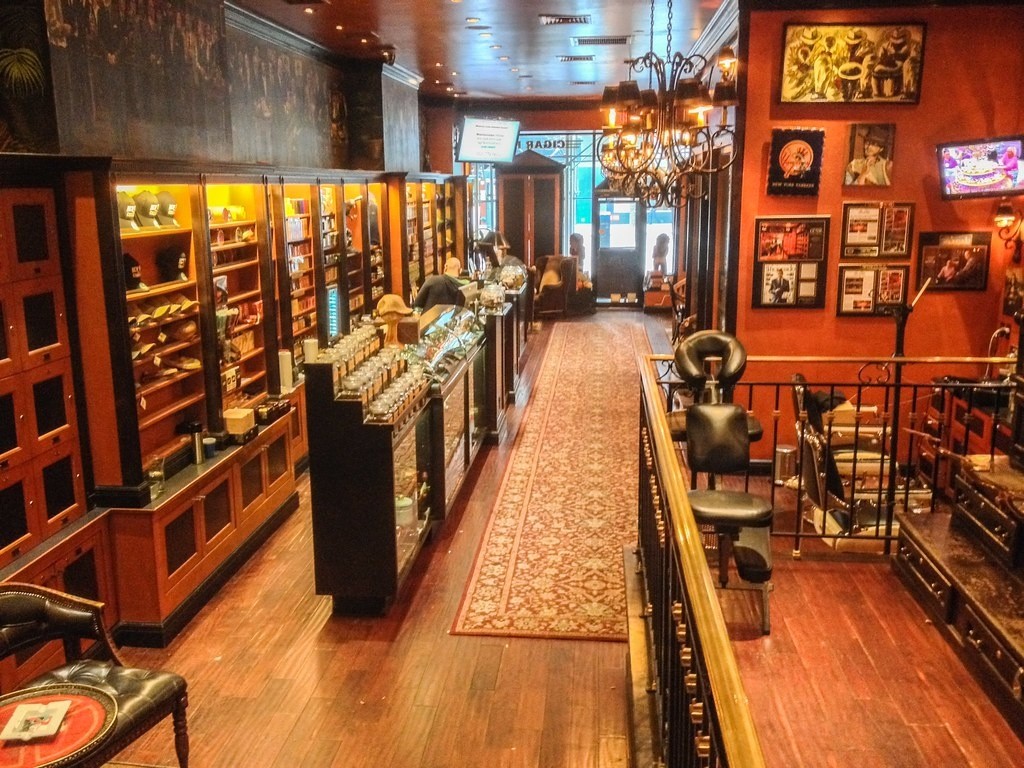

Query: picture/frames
[[751, 214, 831, 310]]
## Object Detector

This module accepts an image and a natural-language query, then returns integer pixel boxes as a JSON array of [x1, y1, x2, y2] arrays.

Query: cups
[[147, 454, 165, 490], [190, 421, 203, 465], [395, 494, 413, 526], [203, 437, 217, 457]]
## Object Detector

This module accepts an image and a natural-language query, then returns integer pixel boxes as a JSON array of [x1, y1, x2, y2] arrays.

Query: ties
[[778, 278, 781, 288]]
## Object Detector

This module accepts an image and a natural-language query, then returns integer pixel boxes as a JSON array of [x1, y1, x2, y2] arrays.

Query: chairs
[[533, 254, 578, 321], [672, 327, 775, 635], [0, 580, 192, 768], [788, 372, 932, 562]]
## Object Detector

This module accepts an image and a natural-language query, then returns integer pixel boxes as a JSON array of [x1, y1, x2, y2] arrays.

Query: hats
[[132, 321, 197, 360], [377, 294, 413, 316], [213, 275, 228, 295], [117, 190, 178, 228], [127, 291, 200, 324]]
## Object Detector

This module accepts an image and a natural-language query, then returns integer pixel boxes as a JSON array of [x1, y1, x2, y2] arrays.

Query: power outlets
[[1005, 324, 1012, 337]]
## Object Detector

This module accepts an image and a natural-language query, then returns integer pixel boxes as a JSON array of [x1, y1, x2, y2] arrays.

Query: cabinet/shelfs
[[57, 154, 479, 658], [917, 387, 1016, 505]]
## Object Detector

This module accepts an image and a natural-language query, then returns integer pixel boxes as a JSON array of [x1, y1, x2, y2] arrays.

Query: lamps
[[591, 0, 740, 205], [480, 230, 510, 262]]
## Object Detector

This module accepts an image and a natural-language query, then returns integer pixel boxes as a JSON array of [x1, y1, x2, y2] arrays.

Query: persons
[[938, 251, 978, 283], [994, 146, 1018, 187], [944, 149, 957, 188], [769, 268, 789, 303], [844, 125, 893, 186], [414, 257, 470, 317]]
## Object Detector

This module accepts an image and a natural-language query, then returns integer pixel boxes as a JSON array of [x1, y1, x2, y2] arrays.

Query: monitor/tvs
[[454, 116, 521, 164], [935, 134, 1024, 200]]
